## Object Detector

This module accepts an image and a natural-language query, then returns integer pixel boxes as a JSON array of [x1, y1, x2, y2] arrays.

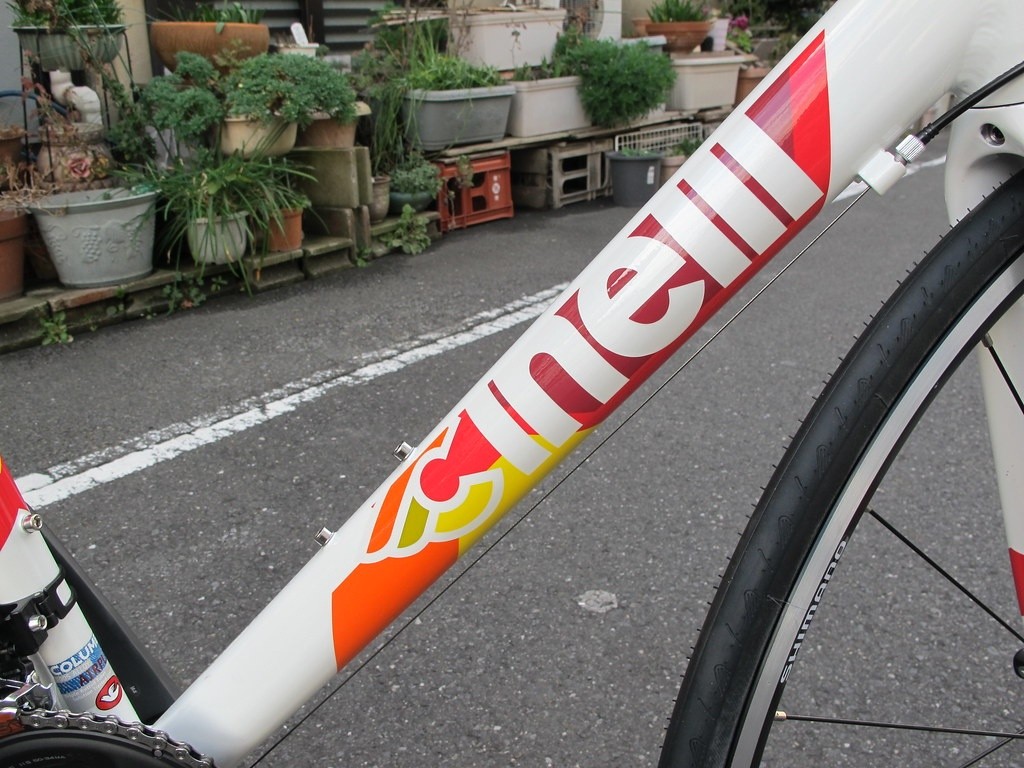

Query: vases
[[736, 64, 770, 101]]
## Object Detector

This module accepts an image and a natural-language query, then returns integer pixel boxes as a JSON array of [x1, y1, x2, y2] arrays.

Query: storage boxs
[[547, 137, 616, 210], [427, 152, 515, 233]]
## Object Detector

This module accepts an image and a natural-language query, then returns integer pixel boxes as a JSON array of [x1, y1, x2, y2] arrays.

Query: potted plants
[[0, 0, 749, 312]]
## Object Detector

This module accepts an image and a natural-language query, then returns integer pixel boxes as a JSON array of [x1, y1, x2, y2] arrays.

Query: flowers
[[704, 6, 761, 68]]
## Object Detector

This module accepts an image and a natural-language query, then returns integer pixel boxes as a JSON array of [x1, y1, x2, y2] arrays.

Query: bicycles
[[0, 0, 1024, 768]]
